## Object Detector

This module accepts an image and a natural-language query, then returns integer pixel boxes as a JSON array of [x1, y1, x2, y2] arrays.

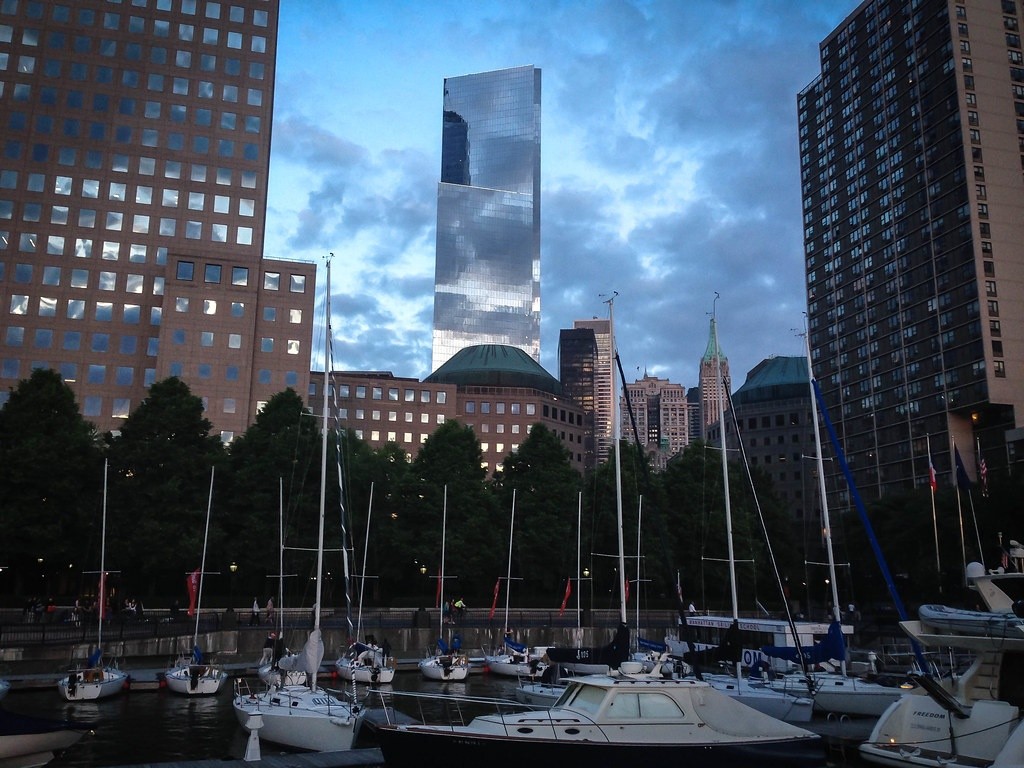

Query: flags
[[980, 448, 988, 479], [928, 464, 936, 491], [954, 445, 969, 485], [490, 581, 500, 619], [186, 568, 199, 616], [625, 577, 629, 602], [559, 577, 571, 616], [1002, 550, 1008, 569], [98, 573, 107, 620]]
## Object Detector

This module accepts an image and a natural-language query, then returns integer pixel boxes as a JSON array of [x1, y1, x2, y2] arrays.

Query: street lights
[[582, 567, 591, 612], [226, 560, 239, 612], [418, 563, 428, 612]]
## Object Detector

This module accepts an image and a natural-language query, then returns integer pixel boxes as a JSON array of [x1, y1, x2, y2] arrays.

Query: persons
[[170, 600, 179, 624], [379, 638, 392, 666], [265, 596, 275, 623], [23, 597, 55, 623], [444, 597, 466, 624], [72, 599, 98, 628], [122, 597, 145, 624], [105, 601, 112, 624], [253, 597, 260, 627], [269, 630, 285, 673]]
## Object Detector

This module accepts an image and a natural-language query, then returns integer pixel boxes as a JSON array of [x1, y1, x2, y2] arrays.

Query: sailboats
[[162, 463, 230, 698], [331, 478, 398, 685], [544, 285, 908, 725], [413, 484, 472, 682], [481, 488, 551, 680], [57, 456, 132, 701], [224, 249, 370, 754], [257, 474, 307, 688]]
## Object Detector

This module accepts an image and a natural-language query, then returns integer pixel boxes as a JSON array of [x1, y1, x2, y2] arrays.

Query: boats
[[808, 370, 1024, 768], [0, 703, 102, 768], [321, 649, 831, 768]]
